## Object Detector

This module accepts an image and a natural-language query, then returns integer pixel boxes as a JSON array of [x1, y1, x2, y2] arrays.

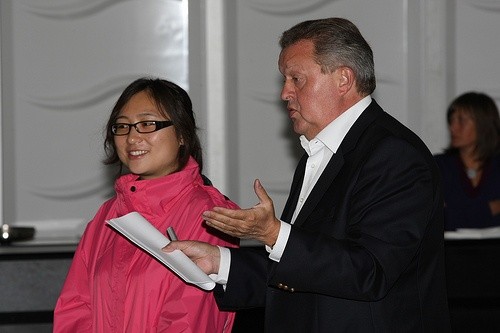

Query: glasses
[[112, 120, 174, 135]]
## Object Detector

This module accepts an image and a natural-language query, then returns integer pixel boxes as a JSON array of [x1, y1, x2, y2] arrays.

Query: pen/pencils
[[165, 225, 178, 244]]
